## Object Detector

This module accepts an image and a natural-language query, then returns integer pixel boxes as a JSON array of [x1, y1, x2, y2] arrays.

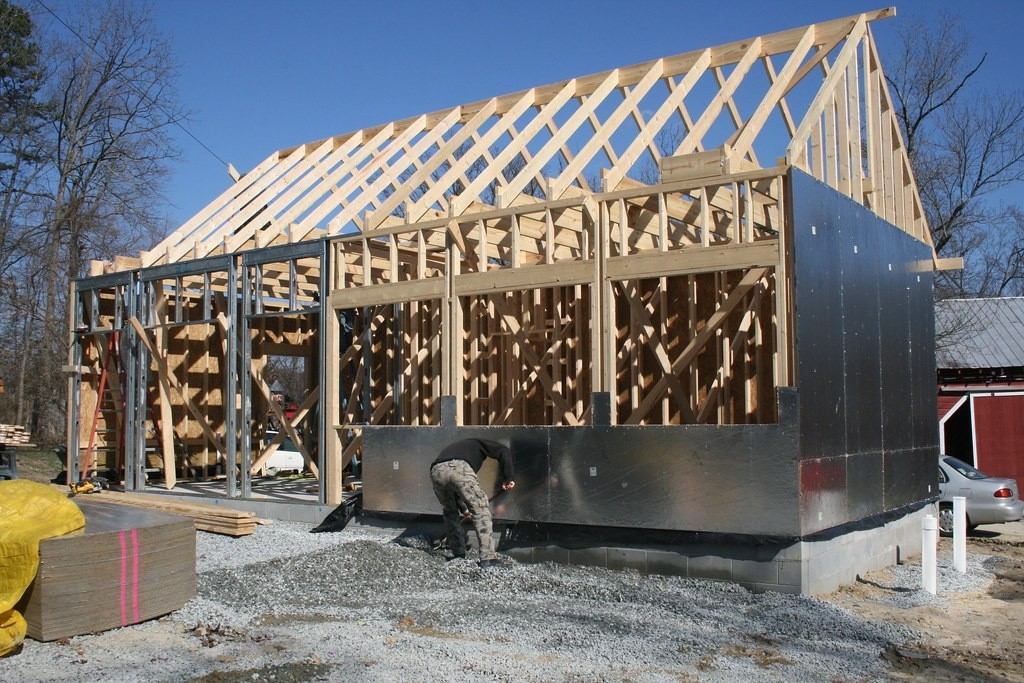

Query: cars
[[262, 428, 305, 478], [937, 453, 1024, 538]]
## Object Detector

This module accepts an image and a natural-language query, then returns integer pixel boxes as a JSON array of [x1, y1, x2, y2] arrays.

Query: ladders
[[80, 331, 166, 483]]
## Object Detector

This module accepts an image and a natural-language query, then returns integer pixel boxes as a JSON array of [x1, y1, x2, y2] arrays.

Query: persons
[[492, 439, 581, 522], [430, 437, 514, 568]]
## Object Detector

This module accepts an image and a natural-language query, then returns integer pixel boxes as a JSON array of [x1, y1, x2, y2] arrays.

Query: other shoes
[[455, 554, 465, 559], [481, 558, 509, 568]]
